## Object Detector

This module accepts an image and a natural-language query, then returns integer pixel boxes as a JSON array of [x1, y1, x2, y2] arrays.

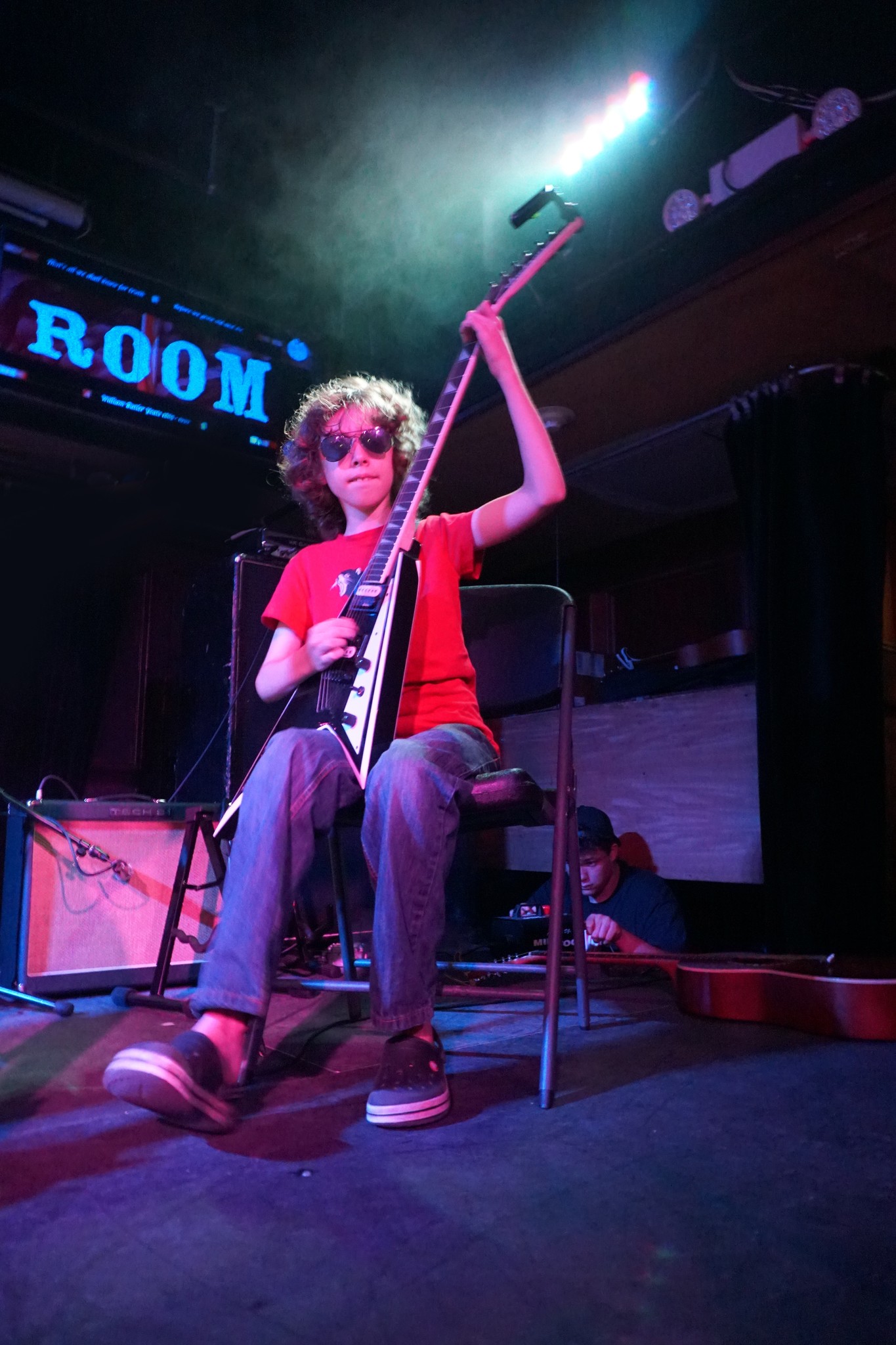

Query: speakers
[[177, 554, 309, 810], [0, 798, 225, 1001]]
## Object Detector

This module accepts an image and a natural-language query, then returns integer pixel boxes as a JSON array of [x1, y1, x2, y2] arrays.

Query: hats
[[564, 805, 623, 857]]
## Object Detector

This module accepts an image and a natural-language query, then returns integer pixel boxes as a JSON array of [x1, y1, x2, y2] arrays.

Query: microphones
[[111, 859, 133, 881]]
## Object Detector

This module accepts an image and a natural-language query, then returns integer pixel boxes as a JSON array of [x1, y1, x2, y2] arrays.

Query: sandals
[[102, 1028, 237, 1134], [366, 1025, 450, 1127]]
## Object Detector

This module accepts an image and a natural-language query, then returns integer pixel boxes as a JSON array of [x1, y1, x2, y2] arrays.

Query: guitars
[[473, 949, 896, 1040], [208, 183, 587, 840]]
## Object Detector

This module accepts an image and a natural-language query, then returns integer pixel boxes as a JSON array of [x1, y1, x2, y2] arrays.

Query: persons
[[102, 300, 566, 1130], [502, 806, 688, 955]]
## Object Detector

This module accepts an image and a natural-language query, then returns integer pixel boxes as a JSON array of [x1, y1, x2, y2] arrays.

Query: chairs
[[314, 582, 588, 1107]]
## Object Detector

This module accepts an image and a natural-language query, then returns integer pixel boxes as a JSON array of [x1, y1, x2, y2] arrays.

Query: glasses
[[316, 427, 393, 461]]
